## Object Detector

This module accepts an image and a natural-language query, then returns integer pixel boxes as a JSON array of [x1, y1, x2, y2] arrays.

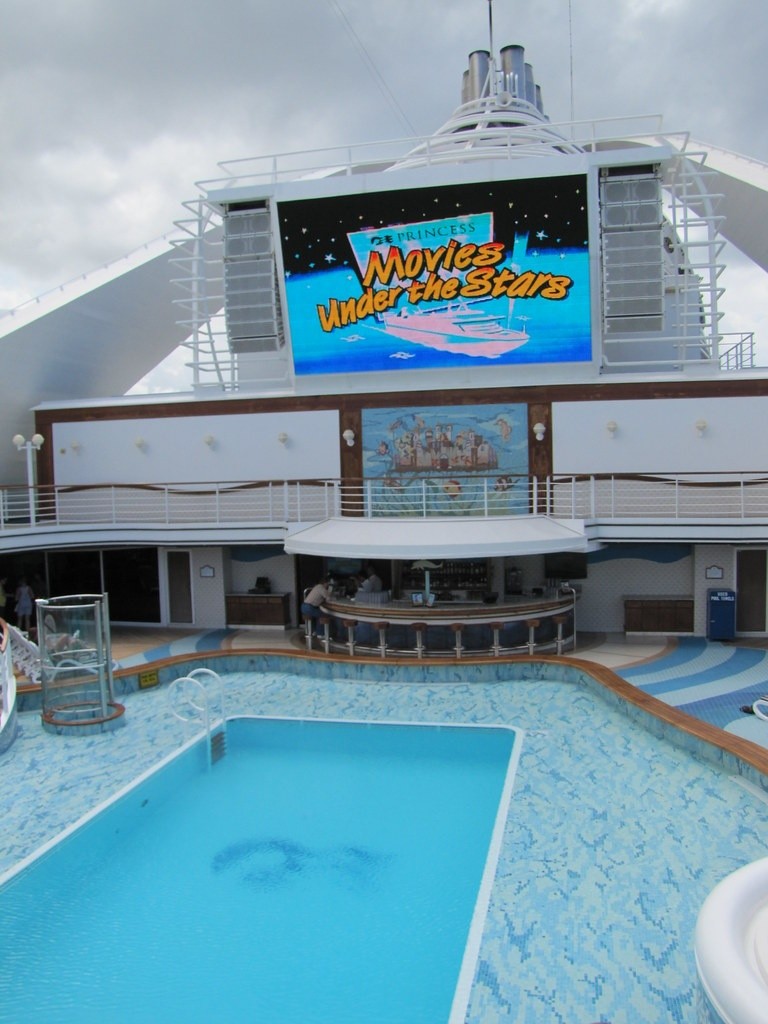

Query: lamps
[[203, 435, 214, 446], [343, 428, 355, 447], [533, 422, 548, 441], [608, 420, 617, 439], [72, 442, 80, 452], [278, 432, 287, 446], [697, 418, 707, 436], [134, 437, 144, 448]]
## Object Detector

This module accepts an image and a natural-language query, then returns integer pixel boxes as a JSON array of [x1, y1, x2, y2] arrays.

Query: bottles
[[355, 588, 391, 605], [402, 560, 489, 589], [506, 567, 523, 593]]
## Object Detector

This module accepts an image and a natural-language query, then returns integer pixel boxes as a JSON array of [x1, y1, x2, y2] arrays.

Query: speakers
[[222, 211, 285, 354], [600, 180, 666, 333]]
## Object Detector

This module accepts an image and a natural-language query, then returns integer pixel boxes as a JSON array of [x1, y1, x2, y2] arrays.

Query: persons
[[301, 576, 334, 639], [349, 564, 382, 593], [14, 578, 35, 635], [0, 576, 8, 619]]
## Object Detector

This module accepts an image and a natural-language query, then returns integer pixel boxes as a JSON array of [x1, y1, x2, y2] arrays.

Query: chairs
[[6, 621, 115, 684]]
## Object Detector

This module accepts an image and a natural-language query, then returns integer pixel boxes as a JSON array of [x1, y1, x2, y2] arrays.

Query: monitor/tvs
[[545, 551, 587, 580]]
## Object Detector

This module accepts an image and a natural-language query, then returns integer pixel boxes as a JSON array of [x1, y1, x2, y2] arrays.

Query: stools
[[318, 616, 330, 653], [490, 622, 504, 655], [410, 623, 428, 658], [526, 618, 541, 655], [449, 622, 466, 658], [552, 615, 569, 655], [343, 620, 358, 655], [302, 615, 315, 648], [375, 621, 390, 657]]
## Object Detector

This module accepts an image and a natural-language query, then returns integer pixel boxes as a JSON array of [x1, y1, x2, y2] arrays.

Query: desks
[[227, 592, 290, 626], [623, 594, 695, 631]]
